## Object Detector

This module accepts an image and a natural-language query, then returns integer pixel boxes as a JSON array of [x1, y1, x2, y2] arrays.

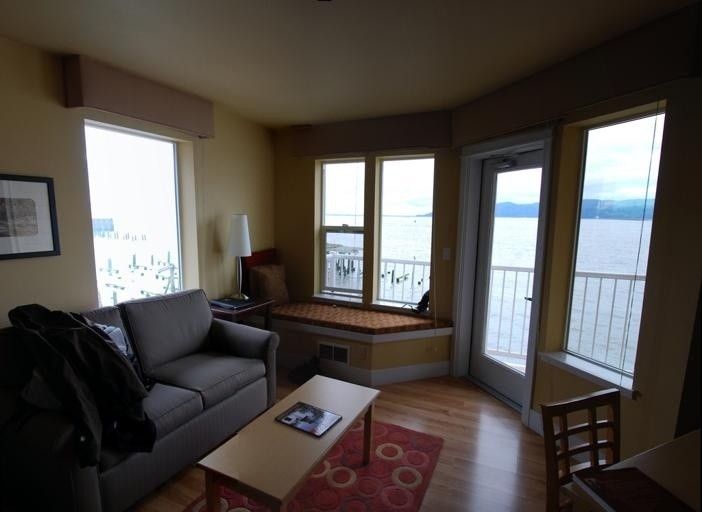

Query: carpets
[[181, 418, 444, 512]]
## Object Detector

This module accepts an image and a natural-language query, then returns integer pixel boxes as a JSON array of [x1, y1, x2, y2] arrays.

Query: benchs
[[237, 248, 454, 387]]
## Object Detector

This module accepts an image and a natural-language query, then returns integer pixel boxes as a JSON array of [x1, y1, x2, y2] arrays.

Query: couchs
[[0, 288, 280, 511]]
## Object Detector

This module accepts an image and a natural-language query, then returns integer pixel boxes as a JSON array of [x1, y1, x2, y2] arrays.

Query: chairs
[[540, 387, 620, 512]]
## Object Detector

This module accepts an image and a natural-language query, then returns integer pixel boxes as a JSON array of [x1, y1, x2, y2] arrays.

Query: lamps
[[226, 214, 252, 301]]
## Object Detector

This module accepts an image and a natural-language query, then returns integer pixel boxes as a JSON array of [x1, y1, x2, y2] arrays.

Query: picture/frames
[[0, 174, 62, 262]]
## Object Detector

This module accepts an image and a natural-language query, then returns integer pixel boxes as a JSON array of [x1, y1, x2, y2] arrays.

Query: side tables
[[210, 294, 276, 330]]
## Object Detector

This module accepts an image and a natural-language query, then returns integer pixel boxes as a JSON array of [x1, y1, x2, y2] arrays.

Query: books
[[276, 401, 342, 438], [574, 467, 695, 512]]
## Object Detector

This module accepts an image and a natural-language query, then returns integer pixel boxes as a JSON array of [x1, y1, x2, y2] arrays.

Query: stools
[[197, 373, 382, 511]]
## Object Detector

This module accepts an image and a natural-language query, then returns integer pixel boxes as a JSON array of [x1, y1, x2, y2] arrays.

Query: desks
[[571, 428, 701, 512]]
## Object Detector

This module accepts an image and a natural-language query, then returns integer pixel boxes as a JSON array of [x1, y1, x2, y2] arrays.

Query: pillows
[[250, 263, 289, 307]]
